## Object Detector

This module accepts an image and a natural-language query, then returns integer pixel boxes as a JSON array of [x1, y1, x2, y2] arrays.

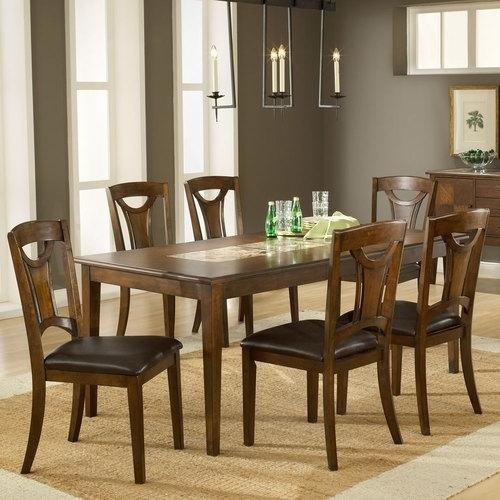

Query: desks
[[82, 229, 473, 457]]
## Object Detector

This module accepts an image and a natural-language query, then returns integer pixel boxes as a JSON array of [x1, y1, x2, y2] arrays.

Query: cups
[[310, 189, 330, 224], [275, 198, 293, 234]]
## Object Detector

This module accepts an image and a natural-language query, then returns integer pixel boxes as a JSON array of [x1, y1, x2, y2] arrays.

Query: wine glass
[[458, 152, 497, 175]]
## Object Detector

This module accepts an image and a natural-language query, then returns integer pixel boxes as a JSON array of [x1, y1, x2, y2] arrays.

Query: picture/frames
[[447, 84, 500, 160]]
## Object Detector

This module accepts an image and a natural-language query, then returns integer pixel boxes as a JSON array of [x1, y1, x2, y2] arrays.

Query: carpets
[[0, 307, 500, 500]]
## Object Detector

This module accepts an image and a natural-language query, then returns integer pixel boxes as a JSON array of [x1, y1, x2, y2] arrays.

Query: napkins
[[304, 209, 361, 241]]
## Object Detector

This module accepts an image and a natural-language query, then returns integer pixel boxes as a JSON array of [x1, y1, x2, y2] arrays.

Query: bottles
[[290, 196, 304, 233], [264, 200, 279, 238]]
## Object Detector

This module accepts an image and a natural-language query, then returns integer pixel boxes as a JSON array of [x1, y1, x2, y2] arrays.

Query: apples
[[463, 149, 494, 164]]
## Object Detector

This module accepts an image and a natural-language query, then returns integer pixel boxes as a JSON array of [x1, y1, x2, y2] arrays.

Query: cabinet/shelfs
[[424, 167, 500, 247]]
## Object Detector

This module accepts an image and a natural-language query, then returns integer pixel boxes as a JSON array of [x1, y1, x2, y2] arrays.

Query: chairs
[[105, 181, 177, 339], [352, 174, 440, 309], [8, 214, 185, 483], [241, 218, 416, 474], [182, 176, 299, 338], [378, 202, 491, 436]]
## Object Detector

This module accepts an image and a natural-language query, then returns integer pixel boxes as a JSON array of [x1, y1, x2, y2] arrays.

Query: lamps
[[206, 0, 346, 122]]
